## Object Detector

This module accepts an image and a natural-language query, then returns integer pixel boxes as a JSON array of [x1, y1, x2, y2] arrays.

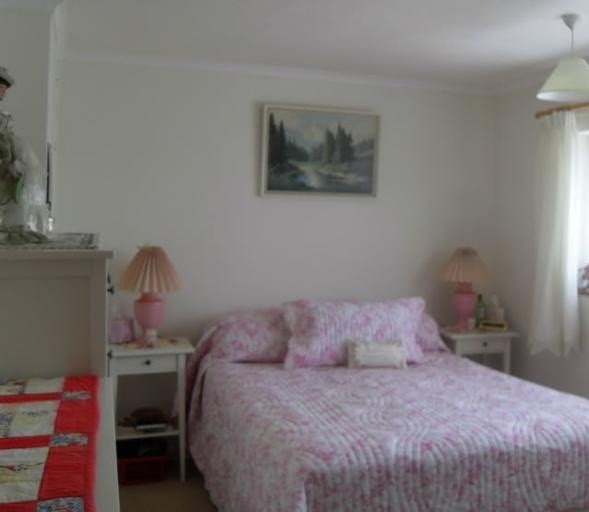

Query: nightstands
[[440, 324, 521, 376], [109, 334, 200, 484]]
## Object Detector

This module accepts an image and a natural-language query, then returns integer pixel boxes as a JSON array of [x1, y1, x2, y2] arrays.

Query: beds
[[187, 293, 588, 510]]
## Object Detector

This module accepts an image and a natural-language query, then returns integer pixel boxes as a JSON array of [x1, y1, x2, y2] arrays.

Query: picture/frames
[[260, 101, 385, 202]]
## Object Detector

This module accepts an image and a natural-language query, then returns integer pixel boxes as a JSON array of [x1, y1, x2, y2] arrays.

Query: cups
[[26, 203, 50, 237], [109, 318, 134, 344]]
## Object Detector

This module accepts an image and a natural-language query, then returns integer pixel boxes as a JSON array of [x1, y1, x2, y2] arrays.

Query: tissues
[[109, 304, 134, 344], [487, 295, 504, 321]]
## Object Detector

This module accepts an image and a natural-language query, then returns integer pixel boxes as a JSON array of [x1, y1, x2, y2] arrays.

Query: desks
[[0, 376, 121, 511]]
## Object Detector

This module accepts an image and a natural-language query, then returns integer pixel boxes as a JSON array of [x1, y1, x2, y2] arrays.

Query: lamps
[[537, 12, 587, 107], [439, 247, 492, 331], [118, 243, 184, 349]]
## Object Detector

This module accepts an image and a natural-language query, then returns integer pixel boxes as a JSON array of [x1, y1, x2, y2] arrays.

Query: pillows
[[280, 294, 427, 370], [344, 337, 409, 369]]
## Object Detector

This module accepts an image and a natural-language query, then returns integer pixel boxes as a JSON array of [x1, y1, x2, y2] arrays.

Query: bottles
[[476, 295, 485, 323]]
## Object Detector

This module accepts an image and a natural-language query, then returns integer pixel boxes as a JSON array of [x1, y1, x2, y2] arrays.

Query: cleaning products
[[475, 294, 486, 321]]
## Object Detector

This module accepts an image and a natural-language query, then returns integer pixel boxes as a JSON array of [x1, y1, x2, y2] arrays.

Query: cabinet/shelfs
[[0, 245, 116, 376]]
